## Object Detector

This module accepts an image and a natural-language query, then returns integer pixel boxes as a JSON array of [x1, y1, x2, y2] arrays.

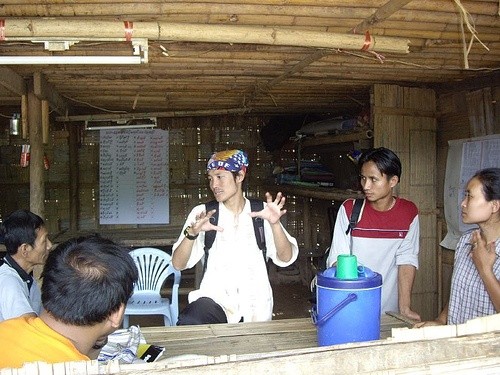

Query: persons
[[169, 150, 299, 326], [414, 167, 500, 328], [326, 147, 422, 320], [0, 208, 138, 368]]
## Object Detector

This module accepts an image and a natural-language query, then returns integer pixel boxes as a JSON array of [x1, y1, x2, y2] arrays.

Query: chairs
[[122, 247, 181, 326]]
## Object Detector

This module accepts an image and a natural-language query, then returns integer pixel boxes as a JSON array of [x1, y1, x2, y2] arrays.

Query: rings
[[472, 242, 477, 248]]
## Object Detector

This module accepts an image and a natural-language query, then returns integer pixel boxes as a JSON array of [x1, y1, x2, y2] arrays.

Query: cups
[[335, 253, 364, 280]]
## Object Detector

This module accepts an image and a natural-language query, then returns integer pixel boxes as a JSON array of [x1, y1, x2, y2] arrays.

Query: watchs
[[184, 226, 199, 240]]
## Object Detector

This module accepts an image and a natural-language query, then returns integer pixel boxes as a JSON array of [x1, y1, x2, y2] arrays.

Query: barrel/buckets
[[310, 267, 384, 346]]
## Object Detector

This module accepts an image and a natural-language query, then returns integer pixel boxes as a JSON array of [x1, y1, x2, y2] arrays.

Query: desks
[[51, 236, 205, 295], [87, 311, 417, 363], [268, 184, 365, 279]]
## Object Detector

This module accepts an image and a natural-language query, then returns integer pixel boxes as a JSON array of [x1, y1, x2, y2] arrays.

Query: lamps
[[87, 124, 155, 130], [0, 56, 144, 64]]
